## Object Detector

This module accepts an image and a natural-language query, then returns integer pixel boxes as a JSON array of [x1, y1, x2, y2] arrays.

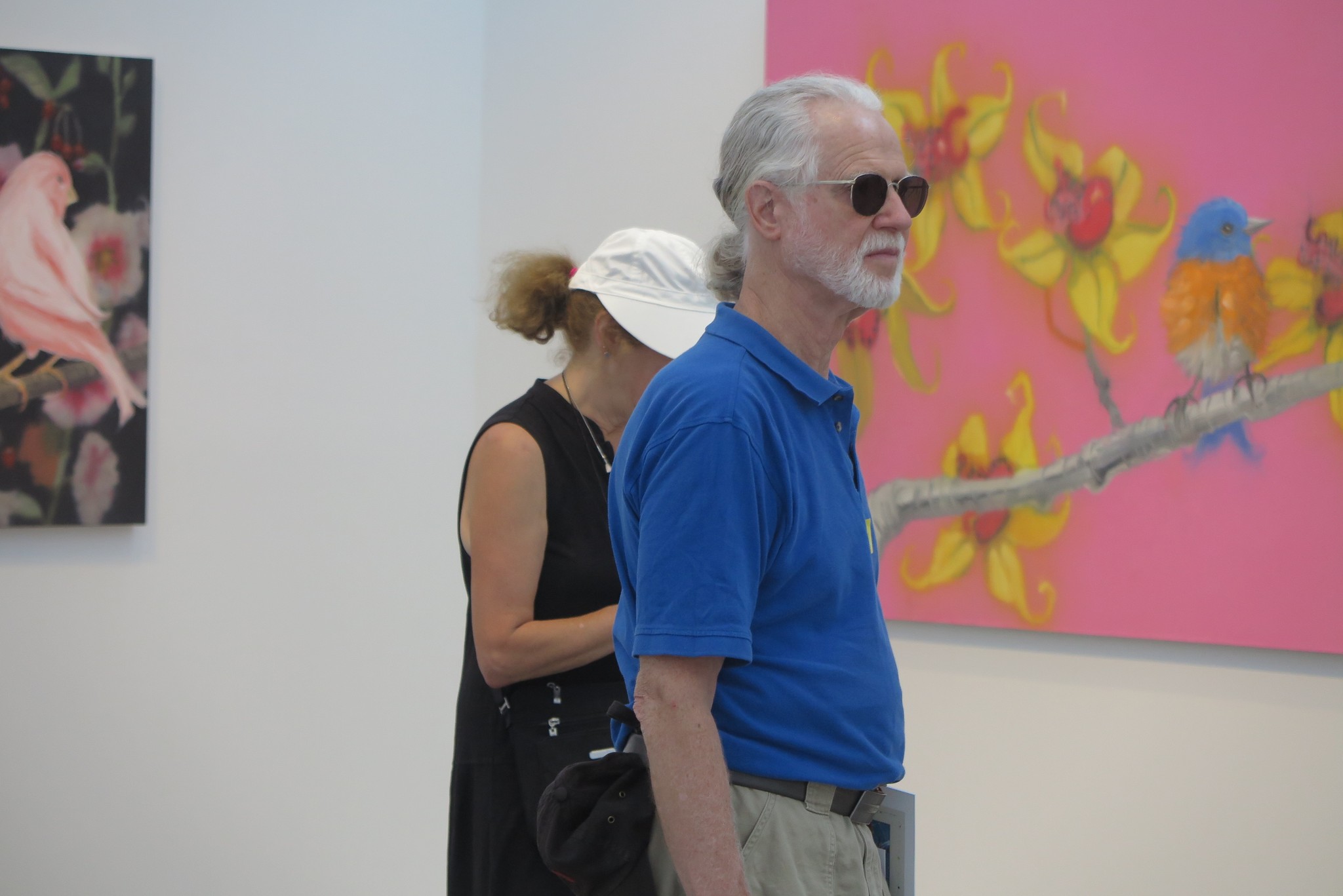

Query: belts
[[728, 765, 887, 826]]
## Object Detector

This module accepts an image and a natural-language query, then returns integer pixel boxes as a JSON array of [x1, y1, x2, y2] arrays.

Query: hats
[[567, 226, 721, 359], [533, 751, 654, 896]]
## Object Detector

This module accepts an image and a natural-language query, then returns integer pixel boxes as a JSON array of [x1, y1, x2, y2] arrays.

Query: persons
[[445, 226, 724, 896], [609, 72, 928, 896]]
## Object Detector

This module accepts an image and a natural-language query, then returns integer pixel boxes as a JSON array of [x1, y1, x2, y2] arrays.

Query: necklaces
[[561, 370, 614, 514]]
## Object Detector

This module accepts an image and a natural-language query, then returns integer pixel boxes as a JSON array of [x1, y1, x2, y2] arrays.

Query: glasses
[[773, 172, 928, 217]]
